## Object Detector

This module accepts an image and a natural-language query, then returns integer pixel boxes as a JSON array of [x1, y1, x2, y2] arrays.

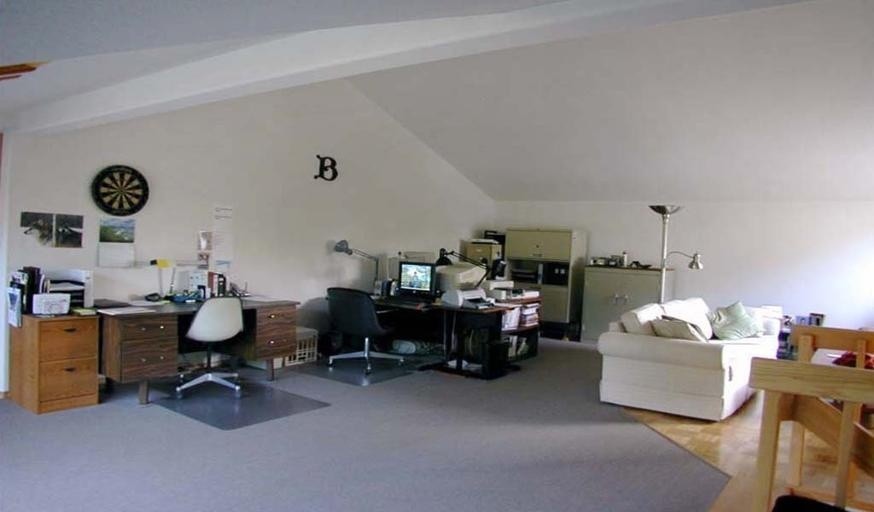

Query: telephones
[[230, 281, 253, 297]]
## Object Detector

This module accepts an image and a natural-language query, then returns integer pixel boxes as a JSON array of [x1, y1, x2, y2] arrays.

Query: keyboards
[[374, 298, 427, 310]]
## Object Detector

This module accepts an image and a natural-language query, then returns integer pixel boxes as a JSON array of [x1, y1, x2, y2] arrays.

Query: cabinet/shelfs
[[8, 312, 101, 414], [503, 225, 588, 342], [465, 243, 501, 278], [580, 266, 674, 349]]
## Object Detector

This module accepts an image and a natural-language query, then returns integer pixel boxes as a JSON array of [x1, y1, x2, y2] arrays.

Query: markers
[[183, 300, 203, 304]]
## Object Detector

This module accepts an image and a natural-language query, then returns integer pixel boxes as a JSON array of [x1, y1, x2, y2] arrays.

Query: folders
[[208, 272, 226, 298], [18, 266, 41, 310]]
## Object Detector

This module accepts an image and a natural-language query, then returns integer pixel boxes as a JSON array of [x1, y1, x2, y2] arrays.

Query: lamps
[[164, 259, 209, 300], [435, 248, 493, 289], [334, 239, 379, 286], [649, 203, 703, 302]]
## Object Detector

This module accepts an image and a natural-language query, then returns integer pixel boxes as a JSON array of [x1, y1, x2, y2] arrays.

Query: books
[[519, 302, 541, 327], [504, 335, 529, 358], [6, 266, 50, 328], [777, 313, 824, 360], [191, 271, 227, 297]]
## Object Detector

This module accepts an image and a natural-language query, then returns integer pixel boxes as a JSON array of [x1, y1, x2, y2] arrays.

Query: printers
[[441, 287, 496, 309]]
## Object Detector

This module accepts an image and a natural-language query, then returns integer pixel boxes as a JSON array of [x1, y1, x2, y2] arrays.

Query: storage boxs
[[245, 326, 319, 370]]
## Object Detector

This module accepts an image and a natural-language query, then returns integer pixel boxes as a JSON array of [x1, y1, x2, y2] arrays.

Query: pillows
[[651, 314, 707, 342], [706, 301, 764, 341]]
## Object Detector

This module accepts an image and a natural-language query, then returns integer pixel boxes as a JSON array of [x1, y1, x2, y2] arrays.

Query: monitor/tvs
[[398, 260, 435, 298]]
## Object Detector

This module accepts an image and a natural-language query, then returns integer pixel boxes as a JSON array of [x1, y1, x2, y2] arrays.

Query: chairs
[[172, 296, 245, 401], [325, 287, 404, 378]]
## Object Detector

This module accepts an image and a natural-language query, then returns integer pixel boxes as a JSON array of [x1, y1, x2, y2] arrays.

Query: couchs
[[596, 297, 782, 423]]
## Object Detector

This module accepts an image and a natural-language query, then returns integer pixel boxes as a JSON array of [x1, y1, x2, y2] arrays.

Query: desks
[[370, 293, 543, 380], [95, 294, 301, 404]]
